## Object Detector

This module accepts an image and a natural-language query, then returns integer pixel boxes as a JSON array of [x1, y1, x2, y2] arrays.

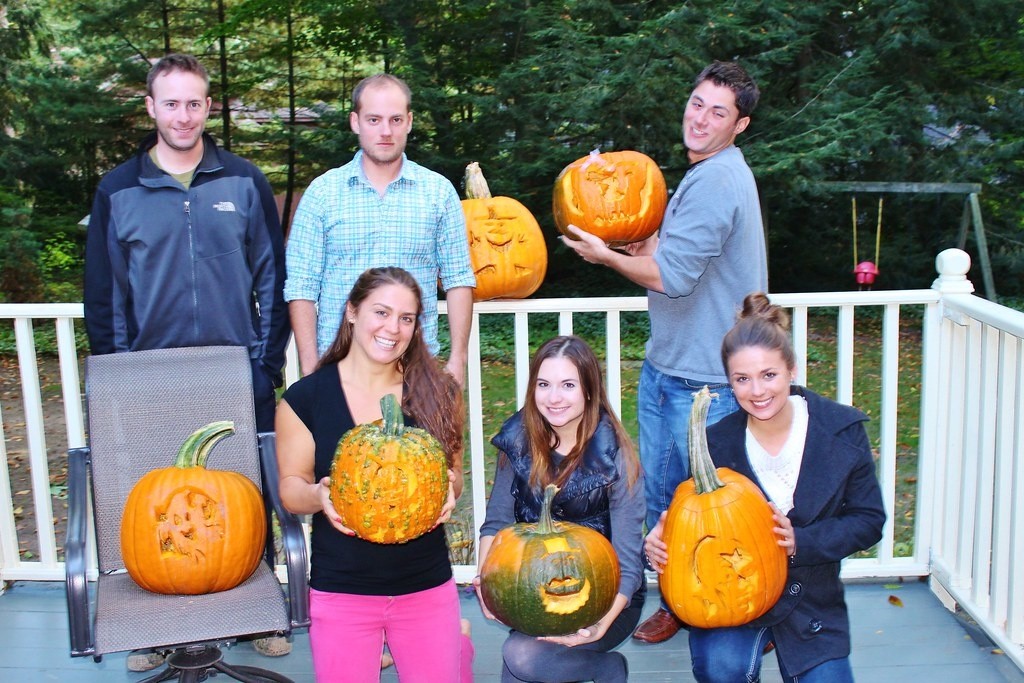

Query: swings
[[851, 196, 883, 285]]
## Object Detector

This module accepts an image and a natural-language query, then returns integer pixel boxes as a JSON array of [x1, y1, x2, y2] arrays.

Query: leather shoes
[[631, 606, 681, 643]]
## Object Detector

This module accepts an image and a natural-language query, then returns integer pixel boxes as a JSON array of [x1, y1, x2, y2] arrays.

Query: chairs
[[64, 346, 311, 683]]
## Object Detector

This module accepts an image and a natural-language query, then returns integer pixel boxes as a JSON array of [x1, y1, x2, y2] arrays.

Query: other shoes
[[254, 636, 292, 657], [127, 649, 172, 671]]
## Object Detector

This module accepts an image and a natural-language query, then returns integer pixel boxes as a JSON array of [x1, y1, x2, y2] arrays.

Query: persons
[[273, 265, 473, 683], [476, 336, 647, 682], [642, 291, 886, 683], [81, 52, 293, 672], [560, 61, 777, 658], [284, 74, 477, 399]]
